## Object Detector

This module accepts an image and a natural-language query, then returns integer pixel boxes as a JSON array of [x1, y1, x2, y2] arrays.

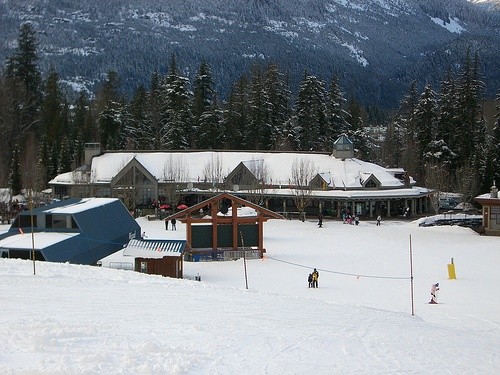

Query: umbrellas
[[177, 204, 188, 210]]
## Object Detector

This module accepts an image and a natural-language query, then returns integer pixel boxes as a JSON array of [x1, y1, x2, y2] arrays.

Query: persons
[[430, 283, 440, 299], [354, 215, 359, 225], [308, 268, 318, 288], [377, 215, 381, 226], [342, 212, 351, 224], [172, 219, 176, 231]]
